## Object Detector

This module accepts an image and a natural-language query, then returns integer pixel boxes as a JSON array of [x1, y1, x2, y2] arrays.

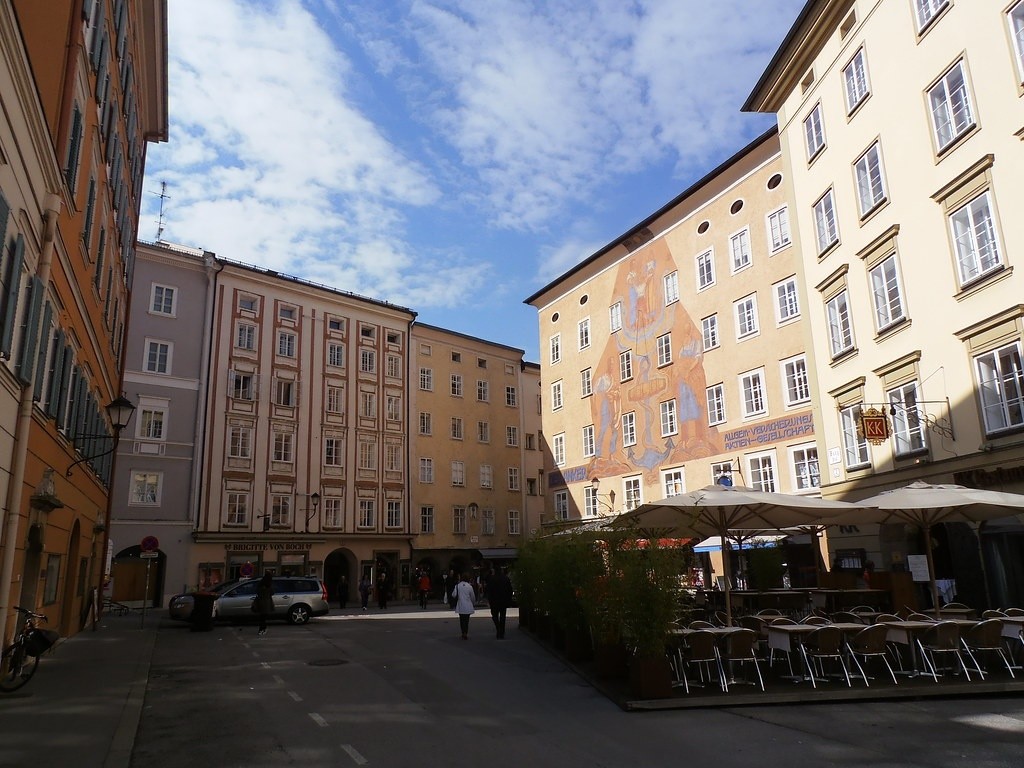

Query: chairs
[[668, 607, 1024, 692]]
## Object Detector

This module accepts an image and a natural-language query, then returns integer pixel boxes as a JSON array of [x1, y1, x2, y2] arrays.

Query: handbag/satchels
[[443, 592, 448, 604], [452, 585, 457, 598], [251, 606, 257, 613]]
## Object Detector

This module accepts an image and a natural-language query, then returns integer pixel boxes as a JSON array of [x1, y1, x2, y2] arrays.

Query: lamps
[[300, 493, 319, 525], [65, 390, 136, 475], [978, 444, 992, 452]]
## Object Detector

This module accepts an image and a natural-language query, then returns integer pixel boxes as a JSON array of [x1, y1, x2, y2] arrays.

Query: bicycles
[[0, 606, 52, 692], [419, 589, 428, 609]]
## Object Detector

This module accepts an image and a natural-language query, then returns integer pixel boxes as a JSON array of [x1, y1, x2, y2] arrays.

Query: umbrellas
[[855, 479, 1024, 620], [600, 484, 877, 680], [538, 517, 691, 578]]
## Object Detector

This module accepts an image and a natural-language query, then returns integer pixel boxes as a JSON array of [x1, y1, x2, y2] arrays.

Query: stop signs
[[240, 563, 254, 576]]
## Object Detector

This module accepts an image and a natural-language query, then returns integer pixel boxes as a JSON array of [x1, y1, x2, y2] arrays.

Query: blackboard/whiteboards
[[83, 587, 99, 621]]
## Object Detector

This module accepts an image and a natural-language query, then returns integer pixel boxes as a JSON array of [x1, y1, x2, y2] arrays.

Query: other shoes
[[380, 605, 384, 609], [497, 629, 501, 640], [259, 629, 268, 635], [384, 606, 387, 609], [365, 608, 368, 611], [499, 635, 504, 640], [463, 633, 467, 640], [363, 607, 365, 610]]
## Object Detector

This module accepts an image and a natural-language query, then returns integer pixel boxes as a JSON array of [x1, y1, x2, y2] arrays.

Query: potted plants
[[509, 512, 694, 695]]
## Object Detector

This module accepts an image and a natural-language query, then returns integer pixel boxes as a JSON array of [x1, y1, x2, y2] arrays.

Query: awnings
[[600, 538, 694, 551], [480, 549, 519, 559], [692, 535, 789, 553]]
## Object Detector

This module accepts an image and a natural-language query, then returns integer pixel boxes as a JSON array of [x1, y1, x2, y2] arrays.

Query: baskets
[[26, 628, 59, 657]]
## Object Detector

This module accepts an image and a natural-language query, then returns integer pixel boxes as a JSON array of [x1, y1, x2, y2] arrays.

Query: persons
[[451, 575, 476, 639], [830, 558, 844, 572], [418, 573, 430, 607], [445, 570, 458, 610], [487, 566, 513, 640], [359, 573, 372, 610], [336, 576, 348, 609], [375, 573, 389, 609], [251, 572, 275, 635]]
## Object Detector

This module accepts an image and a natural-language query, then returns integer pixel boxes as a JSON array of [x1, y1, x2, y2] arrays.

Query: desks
[[987, 616, 1024, 670], [936, 619, 989, 676], [880, 621, 943, 679], [763, 624, 830, 683], [664, 629, 715, 687], [698, 625, 757, 688], [816, 623, 876, 682]]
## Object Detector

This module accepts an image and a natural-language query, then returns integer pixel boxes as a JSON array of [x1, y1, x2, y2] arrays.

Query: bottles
[[863, 571, 872, 589]]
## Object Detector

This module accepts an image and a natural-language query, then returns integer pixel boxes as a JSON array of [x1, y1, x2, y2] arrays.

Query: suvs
[[169, 576, 330, 626]]
